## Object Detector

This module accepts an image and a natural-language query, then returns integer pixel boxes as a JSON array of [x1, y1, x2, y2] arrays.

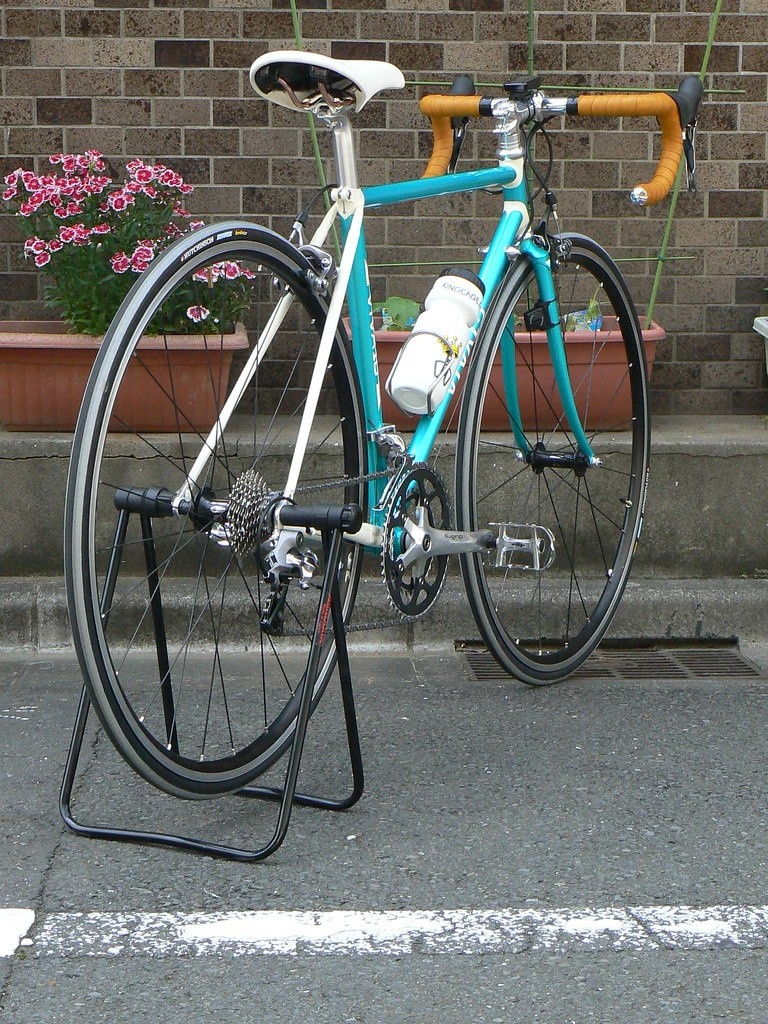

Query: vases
[[0, 325, 246, 431], [342, 314, 667, 433]]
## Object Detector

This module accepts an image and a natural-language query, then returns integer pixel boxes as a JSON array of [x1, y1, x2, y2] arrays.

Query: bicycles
[[54, 48, 706, 863]]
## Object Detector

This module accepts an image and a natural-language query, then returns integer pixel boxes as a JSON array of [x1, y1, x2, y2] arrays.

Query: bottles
[[392, 267, 486, 413]]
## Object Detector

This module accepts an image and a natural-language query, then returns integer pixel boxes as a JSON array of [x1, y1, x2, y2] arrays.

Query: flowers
[[0, 149, 257, 323]]
